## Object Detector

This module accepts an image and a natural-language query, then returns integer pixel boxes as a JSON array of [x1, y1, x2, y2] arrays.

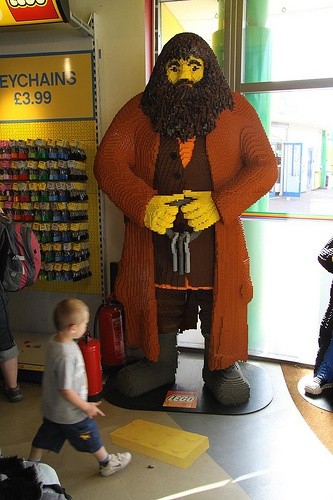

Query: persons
[[29, 299, 132, 479], [304, 335, 333, 395], [0, 208, 24, 402], [94, 32, 277, 409]]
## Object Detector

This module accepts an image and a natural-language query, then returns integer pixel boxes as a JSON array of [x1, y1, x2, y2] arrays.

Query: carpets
[[280, 363, 333, 455]]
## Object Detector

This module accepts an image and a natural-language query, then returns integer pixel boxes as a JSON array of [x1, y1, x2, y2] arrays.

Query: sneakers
[[97, 452, 132, 477]]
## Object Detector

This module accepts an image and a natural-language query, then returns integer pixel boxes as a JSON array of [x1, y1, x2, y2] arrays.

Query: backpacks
[[0, 212, 41, 292]]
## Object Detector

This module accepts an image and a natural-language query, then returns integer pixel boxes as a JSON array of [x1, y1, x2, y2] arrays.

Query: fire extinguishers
[[99, 292, 126, 366], [78, 329, 103, 396]]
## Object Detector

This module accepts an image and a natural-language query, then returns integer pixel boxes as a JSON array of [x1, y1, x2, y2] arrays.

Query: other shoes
[[0, 385, 23, 403]]
